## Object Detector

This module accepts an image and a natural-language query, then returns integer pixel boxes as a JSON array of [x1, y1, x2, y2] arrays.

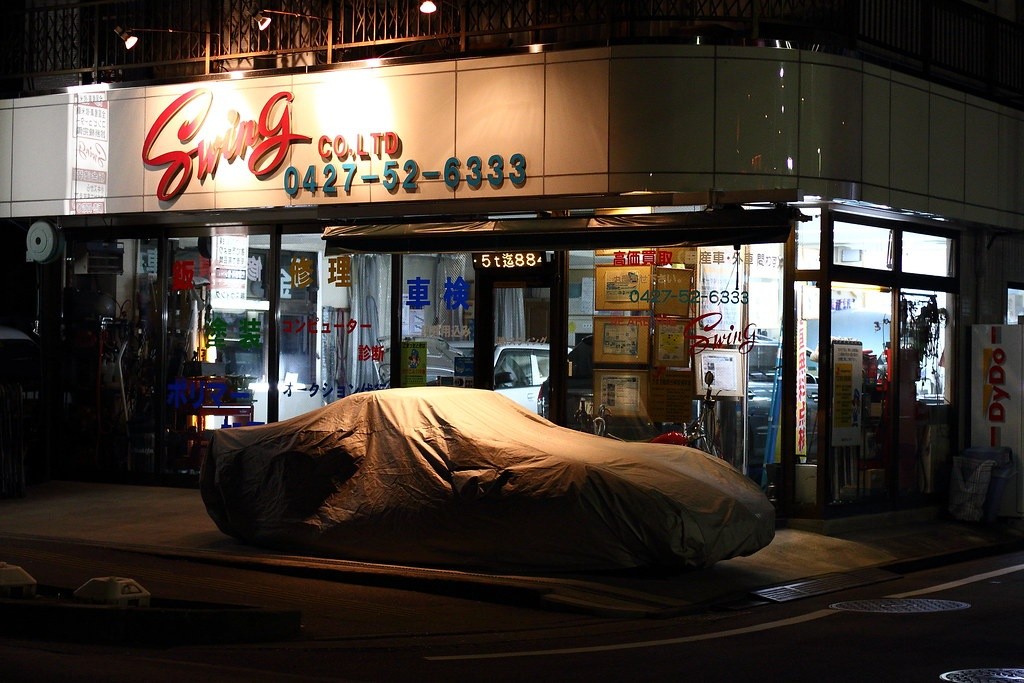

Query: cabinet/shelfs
[[179, 407, 254, 487]]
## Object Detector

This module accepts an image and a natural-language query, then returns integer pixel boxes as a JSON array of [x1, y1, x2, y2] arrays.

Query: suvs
[[734, 334, 830, 438]]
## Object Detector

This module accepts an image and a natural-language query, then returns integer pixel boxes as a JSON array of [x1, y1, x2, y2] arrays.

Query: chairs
[[504, 356, 529, 385]]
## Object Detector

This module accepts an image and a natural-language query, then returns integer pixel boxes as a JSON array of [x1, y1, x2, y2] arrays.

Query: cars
[[201, 384, 774, 574]]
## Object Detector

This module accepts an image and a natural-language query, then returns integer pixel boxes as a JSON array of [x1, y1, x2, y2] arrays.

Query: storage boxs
[[185, 374, 255, 405]]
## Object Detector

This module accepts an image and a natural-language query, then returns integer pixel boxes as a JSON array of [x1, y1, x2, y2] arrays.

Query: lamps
[[72, 244, 125, 276], [114, 26, 220, 49], [245, 8, 332, 31], [420, 0, 437, 13]]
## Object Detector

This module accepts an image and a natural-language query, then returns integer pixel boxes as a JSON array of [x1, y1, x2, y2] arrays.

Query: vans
[[484, 340, 572, 417]]
